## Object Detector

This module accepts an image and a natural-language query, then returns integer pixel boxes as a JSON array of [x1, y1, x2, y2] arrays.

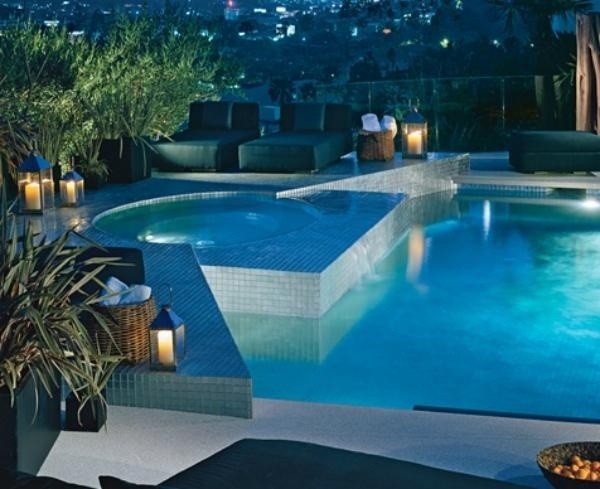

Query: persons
[[382, 47, 401, 77], [348, 49, 383, 82]]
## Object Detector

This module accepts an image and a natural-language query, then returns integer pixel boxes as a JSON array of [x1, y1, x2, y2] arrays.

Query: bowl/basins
[[536, 441, 600, 489]]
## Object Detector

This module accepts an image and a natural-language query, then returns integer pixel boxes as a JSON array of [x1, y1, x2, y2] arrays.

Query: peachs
[[551, 455, 600, 481]]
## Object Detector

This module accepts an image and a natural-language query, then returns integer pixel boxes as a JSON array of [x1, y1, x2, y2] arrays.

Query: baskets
[[81, 295, 157, 365], [357, 130, 395, 161]]
